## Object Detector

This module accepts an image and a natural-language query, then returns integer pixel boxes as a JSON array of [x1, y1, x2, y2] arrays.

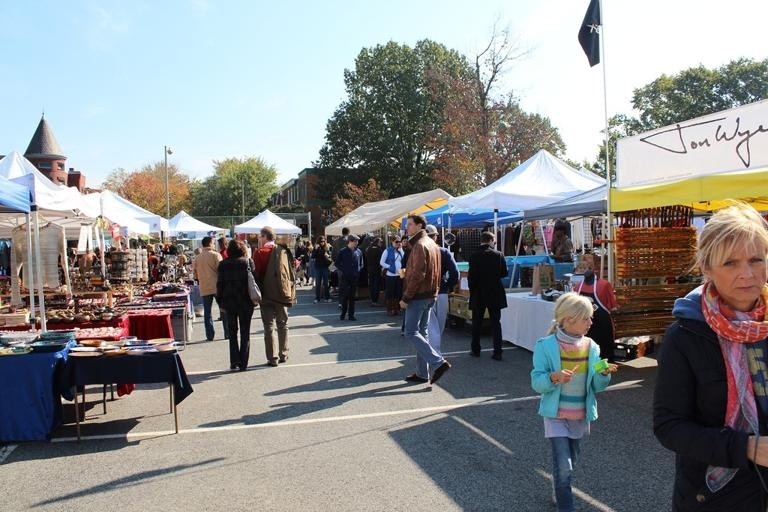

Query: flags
[[577, 0, 603, 68]]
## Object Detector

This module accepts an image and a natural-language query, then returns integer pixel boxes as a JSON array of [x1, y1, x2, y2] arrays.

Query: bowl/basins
[[592, 358, 610, 374]]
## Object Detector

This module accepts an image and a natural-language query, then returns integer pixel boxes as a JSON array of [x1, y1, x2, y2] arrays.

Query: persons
[[58, 241, 203, 284], [421, 222, 461, 367], [252, 225, 297, 368], [295, 227, 411, 320], [217, 240, 255, 371], [466, 230, 511, 361], [279, 238, 294, 271], [548, 217, 574, 263], [398, 212, 452, 386], [653, 204, 768, 511], [573, 270, 617, 366], [530, 291, 621, 511]]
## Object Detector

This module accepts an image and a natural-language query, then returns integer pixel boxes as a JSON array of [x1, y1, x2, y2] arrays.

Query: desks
[[500, 292, 557, 353], [0, 289, 194, 445]]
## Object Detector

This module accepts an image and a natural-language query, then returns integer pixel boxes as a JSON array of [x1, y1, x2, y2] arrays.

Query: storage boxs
[[449, 297, 472, 319], [613, 336, 655, 361]]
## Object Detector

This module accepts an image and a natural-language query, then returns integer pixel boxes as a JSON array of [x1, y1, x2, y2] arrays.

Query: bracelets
[[754, 433, 759, 465]]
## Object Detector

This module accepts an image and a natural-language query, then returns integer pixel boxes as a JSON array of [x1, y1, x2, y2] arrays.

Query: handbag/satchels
[[247, 271, 263, 305], [380, 268, 388, 277]]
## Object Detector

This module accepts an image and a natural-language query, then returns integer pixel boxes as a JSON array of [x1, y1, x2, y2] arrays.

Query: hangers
[[19, 212, 50, 229]]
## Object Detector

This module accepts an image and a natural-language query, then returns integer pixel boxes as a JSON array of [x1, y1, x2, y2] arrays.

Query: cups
[[398, 268, 406, 279]]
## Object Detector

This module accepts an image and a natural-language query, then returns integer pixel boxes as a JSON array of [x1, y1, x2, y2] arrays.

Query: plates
[[0, 327, 174, 358]]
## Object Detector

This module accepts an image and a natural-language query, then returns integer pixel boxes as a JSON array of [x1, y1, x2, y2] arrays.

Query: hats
[[348, 234, 360, 241], [425, 225, 440, 236]]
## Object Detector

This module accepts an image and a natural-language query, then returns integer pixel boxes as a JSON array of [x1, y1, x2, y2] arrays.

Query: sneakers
[[406, 361, 451, 384]]
